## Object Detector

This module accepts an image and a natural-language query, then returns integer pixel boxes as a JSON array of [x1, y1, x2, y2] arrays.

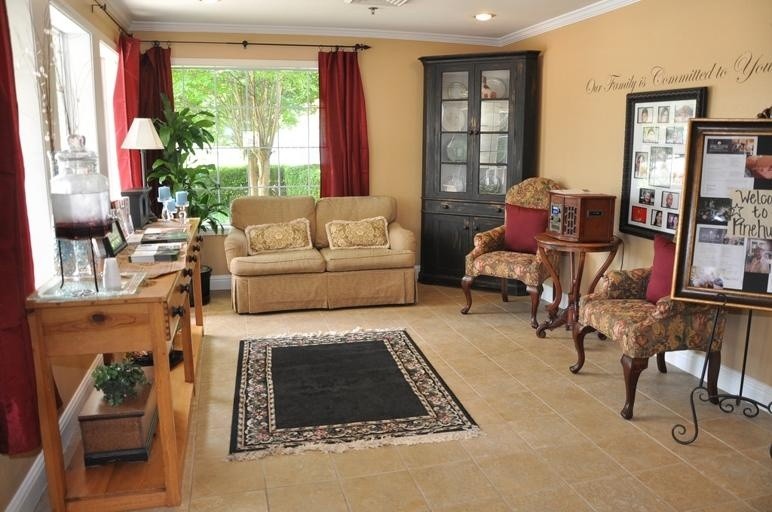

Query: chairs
[[570, 233, 727, 421], [460, 178, 563, 329]]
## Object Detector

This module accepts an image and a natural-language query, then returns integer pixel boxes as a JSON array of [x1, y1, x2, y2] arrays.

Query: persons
[[642, 108, 649, 122], [672, 215, 678, 229], [660, 107, 669, 122], [746, 245, 769, 274], [667, 214, 672, 229], [666, 193, 673, 208], [653, 211, 662, 227]]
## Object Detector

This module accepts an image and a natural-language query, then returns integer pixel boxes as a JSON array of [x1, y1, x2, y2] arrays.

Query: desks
[[28, 218, 204, 512]]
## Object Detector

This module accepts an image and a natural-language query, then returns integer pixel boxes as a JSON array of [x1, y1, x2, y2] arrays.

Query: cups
[[103, 257, 121, 293]]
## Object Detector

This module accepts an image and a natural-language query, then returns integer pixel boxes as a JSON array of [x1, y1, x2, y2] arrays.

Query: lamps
[[476, 14, 494, 23], [121, 116, 164, 190]]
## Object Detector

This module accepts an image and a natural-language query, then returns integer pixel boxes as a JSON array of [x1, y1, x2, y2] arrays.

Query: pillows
[[245, 217, 314, 256], [644, 231, 679, 308], [503, 203, 548, 255], [325, 216, 390, 249]]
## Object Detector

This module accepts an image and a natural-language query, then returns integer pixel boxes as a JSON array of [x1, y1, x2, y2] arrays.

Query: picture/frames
[[617, 88, 707, 242], [672, 118, 772, 309]]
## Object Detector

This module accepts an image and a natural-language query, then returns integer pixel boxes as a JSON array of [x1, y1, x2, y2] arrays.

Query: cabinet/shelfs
[[417, 50, 541, 296]]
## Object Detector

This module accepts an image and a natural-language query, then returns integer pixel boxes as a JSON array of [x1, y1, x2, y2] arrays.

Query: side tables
[[531, 234, 622, 330]]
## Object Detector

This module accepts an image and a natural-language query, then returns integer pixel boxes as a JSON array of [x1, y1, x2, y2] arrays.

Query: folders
[[129, 250, 180, 262]]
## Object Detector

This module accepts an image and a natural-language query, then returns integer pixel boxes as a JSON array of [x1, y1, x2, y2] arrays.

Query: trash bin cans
[[188, 265, 212, 307]]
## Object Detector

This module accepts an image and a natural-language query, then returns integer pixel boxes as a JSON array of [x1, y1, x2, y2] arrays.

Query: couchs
[[225, 197, 418, 313]]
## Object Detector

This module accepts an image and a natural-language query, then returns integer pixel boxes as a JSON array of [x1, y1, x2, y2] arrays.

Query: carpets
[[227, 327, 480, 462]]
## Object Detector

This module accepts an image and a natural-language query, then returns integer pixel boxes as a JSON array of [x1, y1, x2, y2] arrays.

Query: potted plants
[[146, 93, 231, 307], [92, 351, 147, 408]]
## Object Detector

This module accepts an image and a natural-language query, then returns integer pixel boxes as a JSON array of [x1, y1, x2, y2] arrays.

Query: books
[[128, 243, 181, 263], [112, 196, 135, 241], [140, 231, 188, 244]]
